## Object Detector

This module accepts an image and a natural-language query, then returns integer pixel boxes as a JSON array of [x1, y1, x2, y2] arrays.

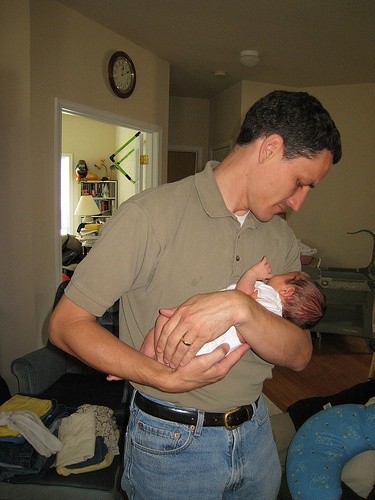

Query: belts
[[134, 390, 260, 431]]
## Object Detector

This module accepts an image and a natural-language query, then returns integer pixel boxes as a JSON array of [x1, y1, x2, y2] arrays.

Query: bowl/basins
[[300, 254, 312, 265]]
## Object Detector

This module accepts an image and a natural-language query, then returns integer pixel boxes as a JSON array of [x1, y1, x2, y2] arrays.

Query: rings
[[180, 336, 193, 347]]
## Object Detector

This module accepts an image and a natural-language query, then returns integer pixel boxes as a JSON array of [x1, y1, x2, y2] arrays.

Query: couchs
[[269, 376, 375, 500], [11, 280, 133, 447], [0, 377, 122, 500]]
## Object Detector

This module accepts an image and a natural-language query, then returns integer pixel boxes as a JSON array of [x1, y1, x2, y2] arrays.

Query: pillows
[[286, 404, 375, 500]]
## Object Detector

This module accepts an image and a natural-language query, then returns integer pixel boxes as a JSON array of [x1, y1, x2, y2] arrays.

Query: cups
[[101, 187, 109, 198]]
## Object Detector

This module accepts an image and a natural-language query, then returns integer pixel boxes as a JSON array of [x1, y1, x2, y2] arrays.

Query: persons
[[106, 256, 327, 382], [48, 90, 342, 496]]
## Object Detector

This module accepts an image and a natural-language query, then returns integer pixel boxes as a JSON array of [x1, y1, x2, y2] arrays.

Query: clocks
[[109, 51, 136, 98]]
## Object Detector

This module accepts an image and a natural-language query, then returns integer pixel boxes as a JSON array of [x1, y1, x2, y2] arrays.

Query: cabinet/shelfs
[[80, 180, 118, 224], [302, 229, 375, 355]]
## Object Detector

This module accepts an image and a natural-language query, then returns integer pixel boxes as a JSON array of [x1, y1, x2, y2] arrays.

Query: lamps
[[74, 195, 100, 222], [95, 159, 110, 180], [240, 50, 259, 68]]
